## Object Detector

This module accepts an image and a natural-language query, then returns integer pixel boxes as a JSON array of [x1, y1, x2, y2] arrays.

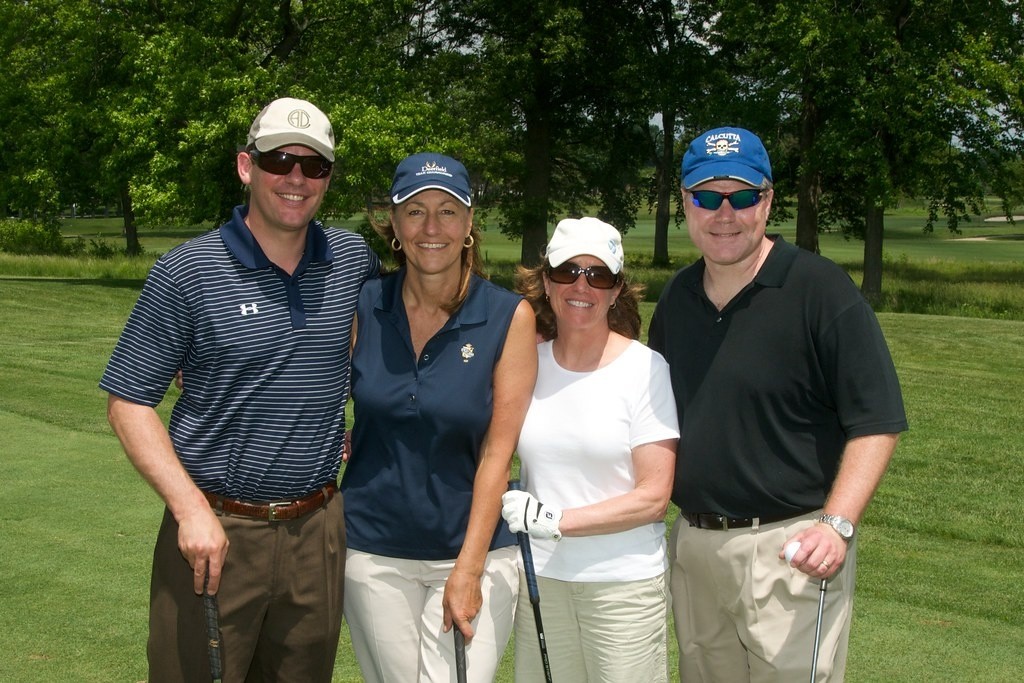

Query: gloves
[[501, 490, 562, 541]]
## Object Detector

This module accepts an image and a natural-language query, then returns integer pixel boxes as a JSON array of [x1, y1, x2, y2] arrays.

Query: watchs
[[816, 509, 857, 544]]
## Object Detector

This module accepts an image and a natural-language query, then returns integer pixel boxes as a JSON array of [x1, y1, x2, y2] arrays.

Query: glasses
[[687, 189, 767, 210], [257, 148, 332, 179], [549, 260, 619, 290]]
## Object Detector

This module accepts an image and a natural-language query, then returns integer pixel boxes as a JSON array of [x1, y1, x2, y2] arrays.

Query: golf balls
[[783, 541, 802, 562]]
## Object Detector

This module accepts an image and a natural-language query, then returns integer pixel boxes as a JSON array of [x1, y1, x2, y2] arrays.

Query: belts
[[203, 483, 334, 522], [681, 506, 822, 532]]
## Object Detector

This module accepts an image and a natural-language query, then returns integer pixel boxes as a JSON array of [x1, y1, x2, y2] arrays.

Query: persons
[[340, 152, 540, 683], [500, 214, 680, 681], [97, 96, 390, 682], [646, 126, 909, 680]]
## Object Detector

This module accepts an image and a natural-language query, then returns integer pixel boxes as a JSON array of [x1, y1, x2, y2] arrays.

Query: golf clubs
[[453, 622, 467, 683], [808, 578, 827, 683], [506, 477, 554, 683], [202, 569, 224, 683]]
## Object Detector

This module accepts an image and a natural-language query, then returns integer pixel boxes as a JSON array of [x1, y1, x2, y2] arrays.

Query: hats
[[680, 126, 773, 189], [545, 216, 624, 275], [245, 96, 336, 162], [391, 153, 471, 207]]
